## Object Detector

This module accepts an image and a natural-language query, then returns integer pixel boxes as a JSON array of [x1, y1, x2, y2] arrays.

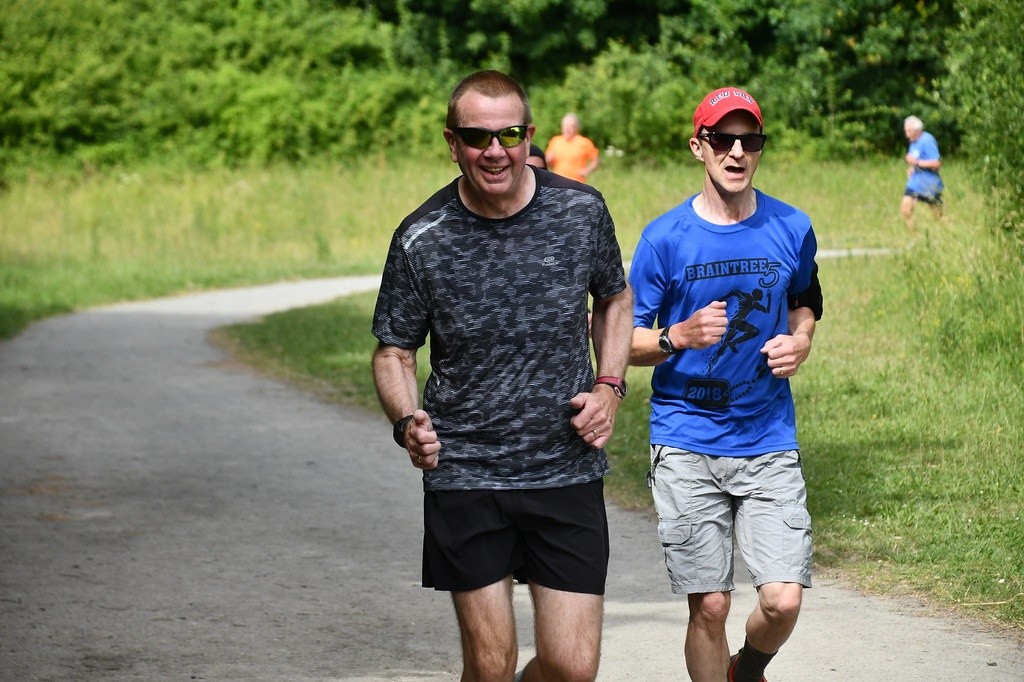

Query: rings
[[780, 367, 784, 375], [592, 429, 598, 439], [416, 454, 422, 464]]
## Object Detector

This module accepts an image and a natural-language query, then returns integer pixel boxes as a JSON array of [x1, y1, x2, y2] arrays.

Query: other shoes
[[726, 647, 767, 682]]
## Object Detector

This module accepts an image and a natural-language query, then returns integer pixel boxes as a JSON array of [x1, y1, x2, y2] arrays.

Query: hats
[[693, 87, 763, 138], [531, 143, 545, 160]]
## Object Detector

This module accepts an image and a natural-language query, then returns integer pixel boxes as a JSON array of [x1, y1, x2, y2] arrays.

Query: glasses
[[449, 125, 528, 149], [698, 132, 767, 152]]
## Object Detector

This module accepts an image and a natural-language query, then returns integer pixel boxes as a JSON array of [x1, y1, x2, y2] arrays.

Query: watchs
[[594, 375, 629, 399], [659, 324, 678, 354], [393, 415, 414, 449]]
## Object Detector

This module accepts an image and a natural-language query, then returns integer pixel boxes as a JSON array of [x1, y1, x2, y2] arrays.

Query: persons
[[371, 69, 635, 682], [626, 87, 822, 682], [527, 112, 600, 338], [901, 115, 944, 235]]
